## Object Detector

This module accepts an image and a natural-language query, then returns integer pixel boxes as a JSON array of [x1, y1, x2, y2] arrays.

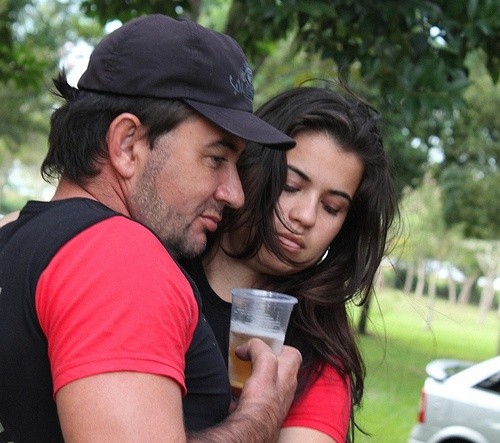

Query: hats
[[76, 11, 296, 151]]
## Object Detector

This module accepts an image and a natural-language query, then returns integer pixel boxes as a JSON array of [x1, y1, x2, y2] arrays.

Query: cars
[[407, 354, 500, 443]]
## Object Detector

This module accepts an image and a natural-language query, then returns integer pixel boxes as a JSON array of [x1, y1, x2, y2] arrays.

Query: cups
[[227, 287, 299, 388]]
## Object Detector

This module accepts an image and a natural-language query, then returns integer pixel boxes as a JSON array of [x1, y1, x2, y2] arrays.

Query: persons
[[0, 16, 305, 443], [175, 74, 401, 443]]
